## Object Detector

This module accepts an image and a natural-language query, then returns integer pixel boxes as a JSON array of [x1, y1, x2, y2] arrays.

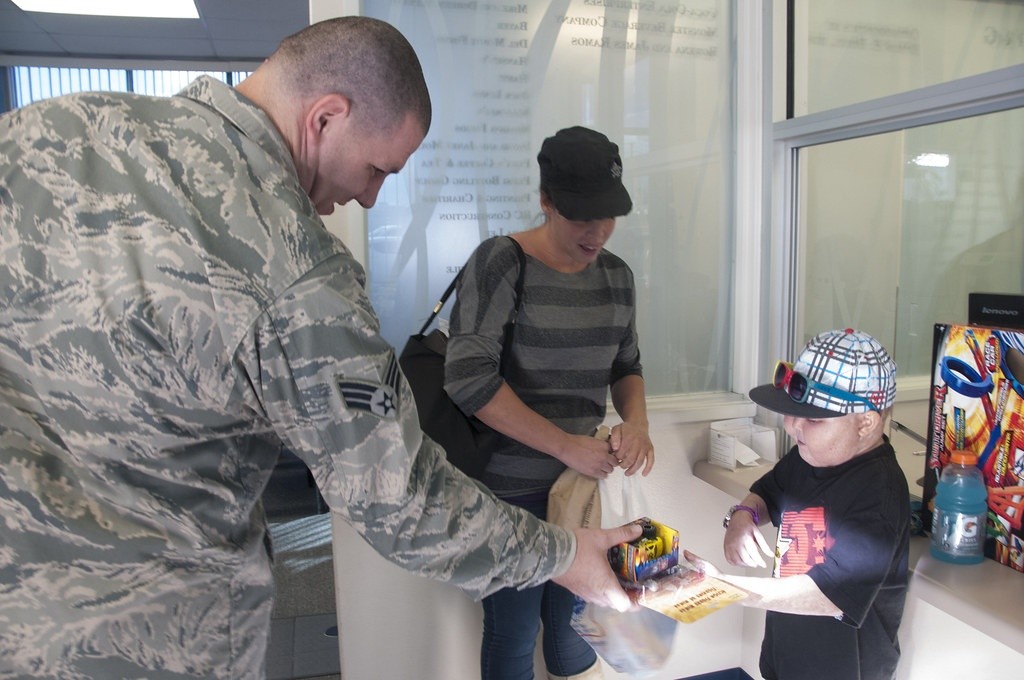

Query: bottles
[[929, 450, 988, 564]]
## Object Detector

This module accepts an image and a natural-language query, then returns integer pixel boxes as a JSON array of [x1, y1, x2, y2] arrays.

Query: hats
[[538, 126, 634, 219], [749, 328, 897, 418]]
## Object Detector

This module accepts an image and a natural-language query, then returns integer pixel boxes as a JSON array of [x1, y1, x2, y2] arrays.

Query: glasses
[[772, 360, 881, 417]]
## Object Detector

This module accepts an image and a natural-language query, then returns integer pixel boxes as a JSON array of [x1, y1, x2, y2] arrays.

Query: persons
[[441, 123, 655, 679], [682, 327, 913, 679], [0, 14, 648, 677]]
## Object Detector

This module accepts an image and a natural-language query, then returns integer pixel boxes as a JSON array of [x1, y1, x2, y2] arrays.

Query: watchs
[[722, 504, 759, 531]]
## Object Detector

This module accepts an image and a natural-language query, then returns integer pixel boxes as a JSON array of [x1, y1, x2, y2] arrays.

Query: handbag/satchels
[[398, 235, 525, 469]]
